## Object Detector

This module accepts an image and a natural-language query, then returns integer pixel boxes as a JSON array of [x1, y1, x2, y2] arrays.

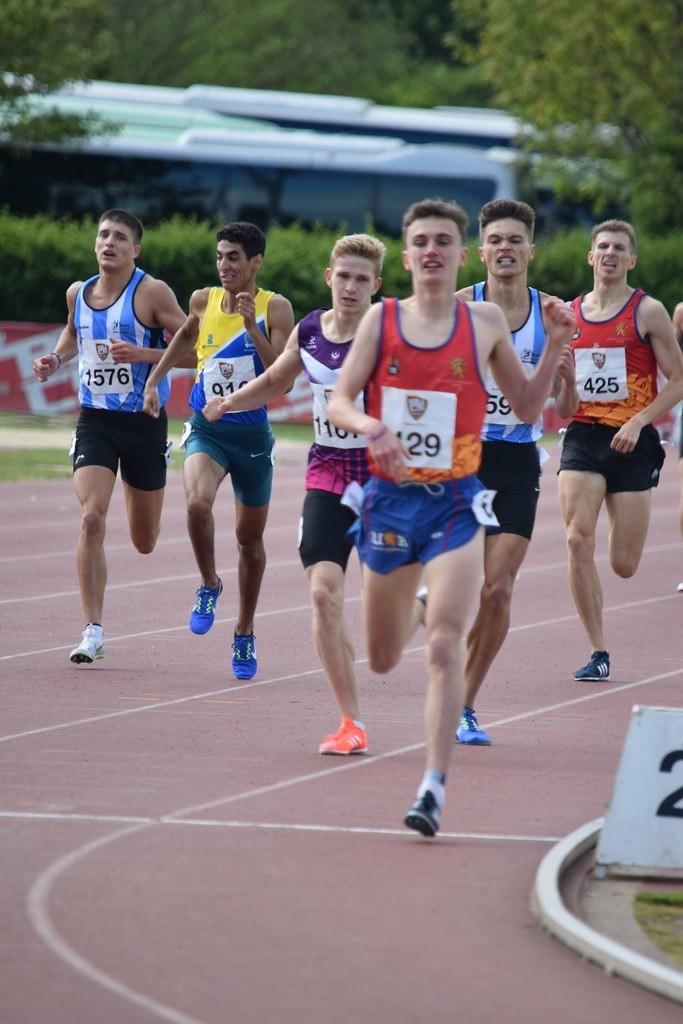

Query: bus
[[0, 73, 631, 246]]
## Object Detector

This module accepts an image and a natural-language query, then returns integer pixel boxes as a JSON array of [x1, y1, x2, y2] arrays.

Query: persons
[[556, 218, 683, 681], [331, 198, 581, 838], [202, 234, 387, 754], [143, 221, 294, 680], [453, 199, 579, 746], [32, 209, 198, 665]]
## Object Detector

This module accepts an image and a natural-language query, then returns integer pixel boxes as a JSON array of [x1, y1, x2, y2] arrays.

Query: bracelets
[[50, 353, 61, 369], [366, 424, 388, 442]]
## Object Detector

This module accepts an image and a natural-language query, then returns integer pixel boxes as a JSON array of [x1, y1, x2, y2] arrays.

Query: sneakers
[[232, 625, 258, 680], [574, 657, 611, 679], [70, 624, 106, 663], [318, 715, 369, 756], [418, 588, 432, 625], [453, 706, 491, 746], [189, 577, 223, 634], [406, 790, 442, 837]]
